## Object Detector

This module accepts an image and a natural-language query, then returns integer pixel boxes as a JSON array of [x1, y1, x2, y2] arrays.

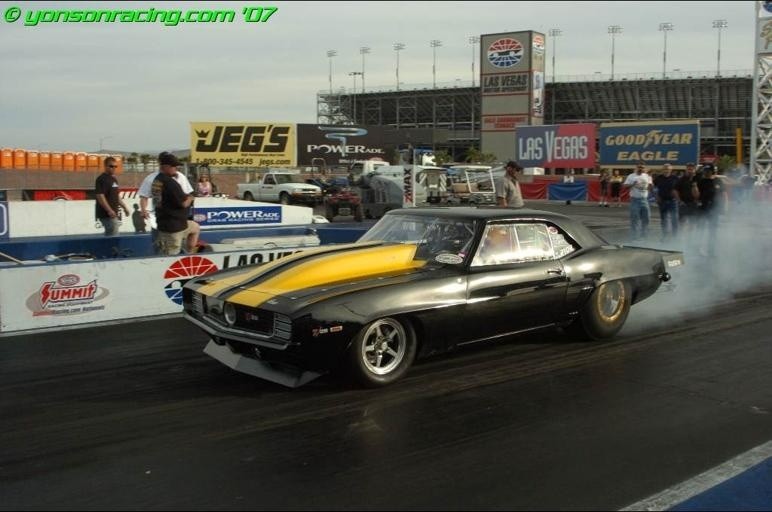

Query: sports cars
[[181, 205, 687, 390]]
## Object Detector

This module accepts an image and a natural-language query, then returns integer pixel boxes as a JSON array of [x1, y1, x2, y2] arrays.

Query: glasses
[[109, 165, 117, 168]]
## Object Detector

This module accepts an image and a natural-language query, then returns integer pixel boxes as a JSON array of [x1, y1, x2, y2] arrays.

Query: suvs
[[325, 175, 365, 223]]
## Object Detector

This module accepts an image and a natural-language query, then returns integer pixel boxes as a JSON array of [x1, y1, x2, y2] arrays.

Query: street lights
[[467, 35, 478, 87], [360, 46, 371, 92], [431, 38, 442, 88], [327, 48, 335, 91], [659, 22, 675, 78], [548, 28, 562, 81], [608, 24, 622, 79], [711, 18, 730, 77], [393, 42, 407, 91]]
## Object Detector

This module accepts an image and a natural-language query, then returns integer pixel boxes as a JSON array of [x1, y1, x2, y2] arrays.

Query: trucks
[[352, 158, 447, 219]]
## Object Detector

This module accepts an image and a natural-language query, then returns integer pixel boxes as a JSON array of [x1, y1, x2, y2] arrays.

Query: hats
[[160, 153, 184, 166], [506, 160, 524, 170]]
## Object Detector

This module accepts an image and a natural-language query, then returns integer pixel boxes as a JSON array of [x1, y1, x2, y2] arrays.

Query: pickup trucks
[[237, 172, 323, 205]]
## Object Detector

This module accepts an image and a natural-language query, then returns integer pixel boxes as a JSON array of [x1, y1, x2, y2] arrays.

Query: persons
[[649, 163, 681, 243], [676, 161, 724, 257], [139, 152, 200, 258], [195, 175, 212, 198], [95, 157, 130, 235], [562, 170, 575, 207], [623, 161, 654, 242], [497, 160, 524, 209], [598, 169, 623, 209]]
